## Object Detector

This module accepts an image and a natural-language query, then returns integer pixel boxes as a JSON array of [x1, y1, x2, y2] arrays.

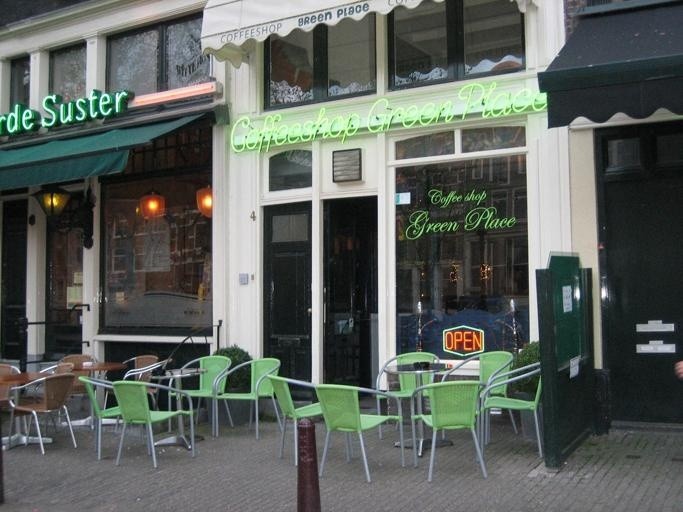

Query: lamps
[[28, 183, 97, 251], [140, 183, 213, 234]]
